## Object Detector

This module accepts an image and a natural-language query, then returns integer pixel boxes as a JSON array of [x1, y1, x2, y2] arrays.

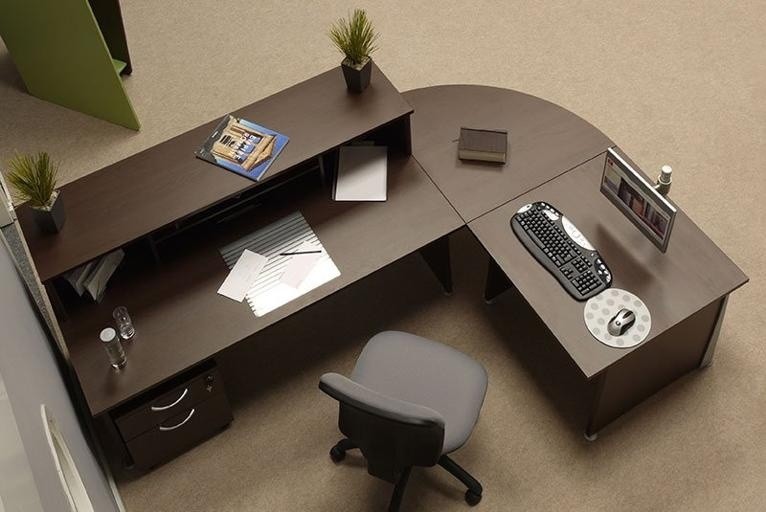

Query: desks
[[57, 153, 467, 471], [467, 145, 750, 441], [14, 55, 415, 325], [398, 84, 618, 225]]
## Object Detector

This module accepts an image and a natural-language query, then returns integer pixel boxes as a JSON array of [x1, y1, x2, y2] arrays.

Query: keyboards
[[509, 200, 613, 303]]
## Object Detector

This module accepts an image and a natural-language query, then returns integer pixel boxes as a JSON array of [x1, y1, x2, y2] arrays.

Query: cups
[[113, 307, 135, 339]]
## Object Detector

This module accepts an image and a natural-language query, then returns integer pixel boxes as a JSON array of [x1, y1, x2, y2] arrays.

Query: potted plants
[[324, 7, 381, 95], [1, 146, 68, 236]]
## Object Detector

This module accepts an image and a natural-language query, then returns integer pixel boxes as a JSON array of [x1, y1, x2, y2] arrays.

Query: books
[[457, 125, 507, 162], [194, 114, 291, 183], [66, 249, 125, 302]]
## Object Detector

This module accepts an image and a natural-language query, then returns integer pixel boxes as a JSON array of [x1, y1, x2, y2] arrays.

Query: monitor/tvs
[[598, 146, 679, 256]]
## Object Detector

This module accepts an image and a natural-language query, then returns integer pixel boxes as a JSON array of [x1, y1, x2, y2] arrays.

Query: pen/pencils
[[279, 251, 323, 256]]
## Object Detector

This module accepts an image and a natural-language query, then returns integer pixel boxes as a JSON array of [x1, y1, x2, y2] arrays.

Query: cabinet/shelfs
[[108, 356, 236, 477]]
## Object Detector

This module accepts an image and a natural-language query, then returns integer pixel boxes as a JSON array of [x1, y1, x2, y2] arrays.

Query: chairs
[[317, 329, 489, 512]]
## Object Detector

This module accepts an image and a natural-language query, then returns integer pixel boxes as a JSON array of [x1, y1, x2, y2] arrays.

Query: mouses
[[607, 308, 636, 337]]
